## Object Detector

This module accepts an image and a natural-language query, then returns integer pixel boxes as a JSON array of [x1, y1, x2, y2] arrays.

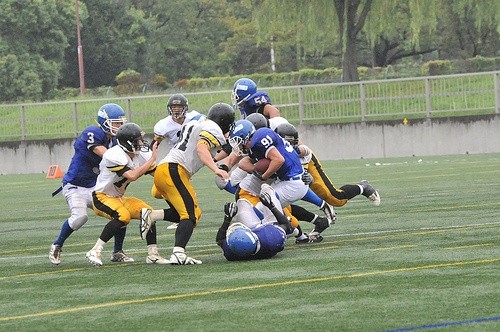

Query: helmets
[[226, 223, 256, 256], [116, 123, 150, 153], [98, 103, 127, 136], [231, 78, 257, 107], [167, 95, 188, 120], [244, 113, 268, 131], [207, 102, 236, 138], [274, 123, 298, 147], [229, 120, 255, 156]]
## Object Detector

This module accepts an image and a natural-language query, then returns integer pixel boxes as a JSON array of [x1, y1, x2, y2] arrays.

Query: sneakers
[[308, 216, 329, 238], [85, 250, 103, 267], [169, 252, 202, 264], [146, 255, 170, 264], [111, 250, 134, 262], [320, 200, 337, 224], [48, 242, 62, 265], [360, 180, 380, 206], [167, 223, 180, 229], [295, 233, 322, 243], [140, 208, 155, 240]]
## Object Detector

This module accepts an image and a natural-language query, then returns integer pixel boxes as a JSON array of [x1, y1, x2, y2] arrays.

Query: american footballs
[[253, 158, 271, 172]]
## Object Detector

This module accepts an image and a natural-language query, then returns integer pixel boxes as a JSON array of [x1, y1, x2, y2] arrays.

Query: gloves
[[259, 191, 275, 210], [224, 202, 238, 218], [303, 172, 312, 185]]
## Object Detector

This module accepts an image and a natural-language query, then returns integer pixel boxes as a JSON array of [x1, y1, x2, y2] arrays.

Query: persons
[[131, 78, 381, 261], [140, 103, 235, 264], [85, 123, 170, 267], [48, 103, 135, 265]]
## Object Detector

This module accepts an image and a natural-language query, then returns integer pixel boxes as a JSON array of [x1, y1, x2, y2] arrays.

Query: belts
[[283, 174, 305, 180], [52, 181, 68, 197]]
